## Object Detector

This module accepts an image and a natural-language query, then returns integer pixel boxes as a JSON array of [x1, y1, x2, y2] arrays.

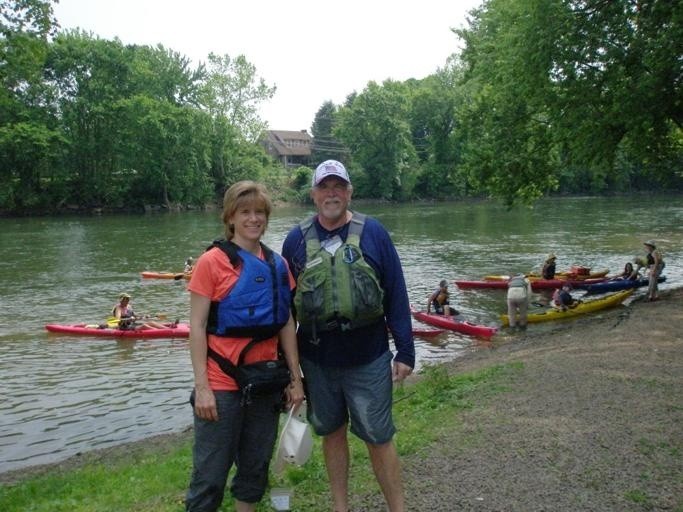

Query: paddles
[[106, 312, 167, 323], [525, 273, 577, 279], [531, 301, 595, 317], [175, 273, 189, 280]]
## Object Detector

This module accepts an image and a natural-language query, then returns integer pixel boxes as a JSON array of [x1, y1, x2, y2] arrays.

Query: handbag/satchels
[[234, 359, 291, 397]]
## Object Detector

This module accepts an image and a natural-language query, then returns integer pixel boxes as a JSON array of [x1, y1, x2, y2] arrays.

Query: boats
[[47, 321, 190, 338], [409, 268, 667, 338], [143, 271, 193, 279]]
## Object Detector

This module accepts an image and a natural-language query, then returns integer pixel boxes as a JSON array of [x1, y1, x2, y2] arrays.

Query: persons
[[633, 257, 665, 279], [427, 280, 459, 316], [188, 178, 305, 512], [111, 293, 179, 331], [507, 272, 532, 327], [643, 240, 663, 302], [280, 158, 416, 511], [184, 256, 194, 274], [609, 262, 642, 282], [552, 287, 572, 306], [542, 253, 557, 280]]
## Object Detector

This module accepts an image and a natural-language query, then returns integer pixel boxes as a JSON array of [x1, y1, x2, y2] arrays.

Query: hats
[[275, 405, 312, 473], [644, 241, 655, 248], [312, 160, 352, 184], [547, 253, 557, 259], [119, 292, 130, 301], [440, 280, 449, 287]]
